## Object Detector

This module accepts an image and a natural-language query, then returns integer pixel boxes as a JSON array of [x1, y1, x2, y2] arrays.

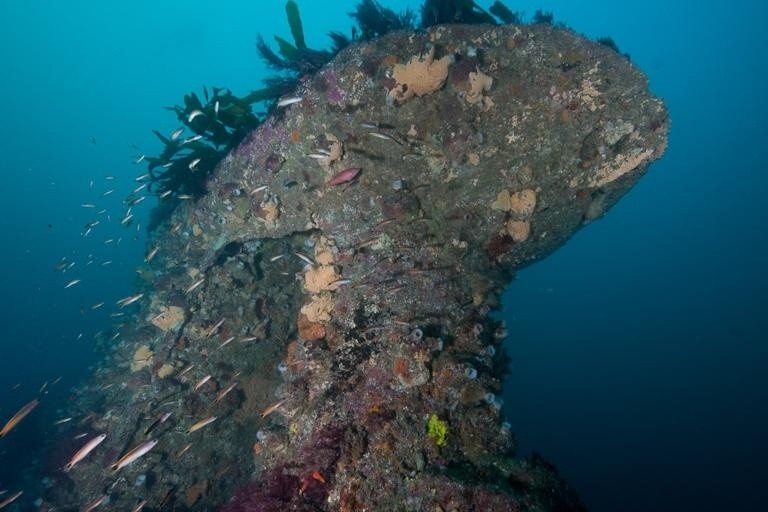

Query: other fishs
[[0, 92, 432, 512]]
